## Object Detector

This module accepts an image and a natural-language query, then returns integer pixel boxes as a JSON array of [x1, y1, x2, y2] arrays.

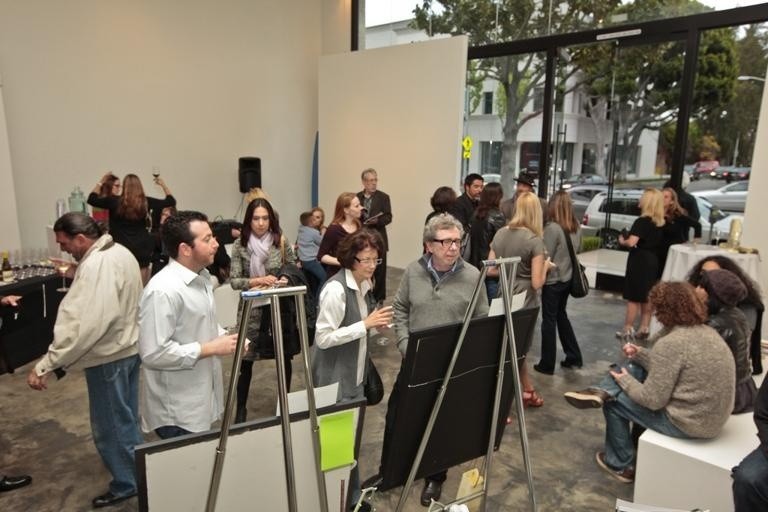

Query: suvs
[[580, 189, 743, 248]]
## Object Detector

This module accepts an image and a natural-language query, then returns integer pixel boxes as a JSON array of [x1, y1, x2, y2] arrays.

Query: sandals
[[518, 387, 546, 410]]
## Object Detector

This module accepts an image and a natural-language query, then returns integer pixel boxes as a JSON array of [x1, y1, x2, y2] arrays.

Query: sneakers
[[595, 450, 636, 484], [635, 325, 648, 340], [615, 324, 636, 340], [564, 387, 607, 410]]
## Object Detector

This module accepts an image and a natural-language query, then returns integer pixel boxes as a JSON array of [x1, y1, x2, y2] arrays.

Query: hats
[[699, 269, 749, 306], [512, 172, 535, 190]]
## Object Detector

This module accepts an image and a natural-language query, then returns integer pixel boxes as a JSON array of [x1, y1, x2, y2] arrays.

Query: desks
[[45, 224, 73, 259]]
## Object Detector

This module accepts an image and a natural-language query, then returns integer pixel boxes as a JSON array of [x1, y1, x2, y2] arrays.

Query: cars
[[691, 181, 748, 211], [561, 176, 610, 187], [692, 161, 720, 174], [730, 168, 751, 178], [560, 186, 614, 222], [711, 166, 735, 181]]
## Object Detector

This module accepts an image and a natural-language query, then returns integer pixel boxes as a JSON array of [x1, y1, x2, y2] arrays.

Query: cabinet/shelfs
[[0, 270, 75, 376]]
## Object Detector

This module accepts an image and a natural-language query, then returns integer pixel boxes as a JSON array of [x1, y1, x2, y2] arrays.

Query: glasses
[[110, 181, 122, 188], [431, 237, 461, 247], [354, 255, 383, 266]]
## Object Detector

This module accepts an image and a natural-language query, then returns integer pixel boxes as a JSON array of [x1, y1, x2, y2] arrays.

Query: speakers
[[238, 156, 262, 194]]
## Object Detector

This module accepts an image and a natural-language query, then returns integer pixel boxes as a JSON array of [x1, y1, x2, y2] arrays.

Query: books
[[357, 206, 384, 223]]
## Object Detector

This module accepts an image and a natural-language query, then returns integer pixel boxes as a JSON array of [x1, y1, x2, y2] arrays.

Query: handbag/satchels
[[364, 354, 385, 406], [562, 227, 589, 299]]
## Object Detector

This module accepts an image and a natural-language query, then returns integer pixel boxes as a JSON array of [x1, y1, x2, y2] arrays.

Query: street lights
[[737, 76, 765, 82]]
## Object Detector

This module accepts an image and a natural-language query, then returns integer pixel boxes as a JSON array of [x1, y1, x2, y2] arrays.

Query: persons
[[317, 191, 364, 279], [346, 168, 393, 306], [310, 208, 328, 236], [691, 255, 764, 375], [230, 187, 280, 238], [423, 186, 457, 256], [534, 189, 589, 375], [360, 211, 493, 507], [298, 227, 395, 512], [503, 171, 538, 221], [156, 206, 177, 227], [483, 192, 558, 424], [296, 211, 328, 286], [563, 281, 736, 483], [138, 210, 249, 439], [631, 269, 759, 447], [468, 182, 507, 305], [87, 170, 177, 286], [616, 187, 666, 340], [27, 212, 145, 508], [92, 175, 122, 236], [661, 188, 703, 243], [730, 371, 768, 512], [231, 196, 298, 424], [0, 275, 32, 492], [457, 173, 485, 221]]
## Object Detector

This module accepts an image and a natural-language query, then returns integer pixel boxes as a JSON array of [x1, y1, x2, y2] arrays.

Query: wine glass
[[713, 229, 722, 248], [151, 166, 160, 185], [56, 251, 70, 292], [620, 328, 637, 369], [224, 328, 242, 377], [13, 247, 56, 280], [375, 300, 392, 346]]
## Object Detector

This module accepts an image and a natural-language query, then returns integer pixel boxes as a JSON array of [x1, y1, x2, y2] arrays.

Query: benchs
[[633, 357, 768, 512]]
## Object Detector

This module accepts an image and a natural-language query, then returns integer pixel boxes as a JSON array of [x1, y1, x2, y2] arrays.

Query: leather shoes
[[0, 473, 34, 493], [533, 361, 555, 377], [361, 475, 393, 493], [559, 358, 586, 370], [92, 490, 139, 508], [420, 481, 441, 506], [233, 406, 249, 428]]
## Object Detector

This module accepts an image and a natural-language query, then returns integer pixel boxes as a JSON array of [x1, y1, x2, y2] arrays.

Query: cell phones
[[607, 363, 622, 374]]
